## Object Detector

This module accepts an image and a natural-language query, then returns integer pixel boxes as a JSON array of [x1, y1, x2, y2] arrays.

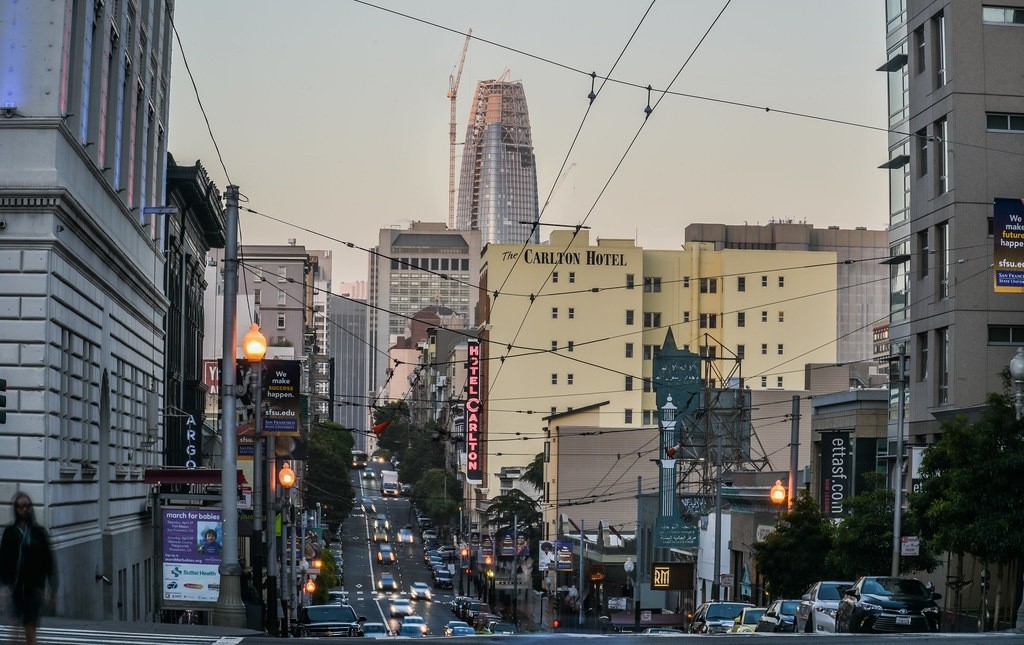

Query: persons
[[517, 535, 529, 555], [540, 542, 554, 565], [197, 529, 222, 555], [0, 492, 58, 645]]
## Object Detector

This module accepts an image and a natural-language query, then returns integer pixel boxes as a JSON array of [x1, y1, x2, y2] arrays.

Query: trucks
[[380, 470, 398, 492]]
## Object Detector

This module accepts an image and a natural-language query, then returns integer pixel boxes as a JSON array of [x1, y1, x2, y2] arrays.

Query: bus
[[351, 450, 368, 469]]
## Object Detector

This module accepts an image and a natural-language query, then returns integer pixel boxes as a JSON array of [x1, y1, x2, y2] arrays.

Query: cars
[[731, 607, 769, 633], [368, 502, 519, 637], [755, 599, 803, 633], [384, 482, 411, 497], [363, 468, 375, 479], [642, 629, 684, 634], [837, 576, 942, 632], [795, 580, 855, 634], [328, 520, 347, 585], [361, 622, 391, 637], [291, 603, 367, 638], [371, 455, 401, 474]]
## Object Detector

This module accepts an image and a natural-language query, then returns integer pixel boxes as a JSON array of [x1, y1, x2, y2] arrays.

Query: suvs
[[687, 599, 755, 633]]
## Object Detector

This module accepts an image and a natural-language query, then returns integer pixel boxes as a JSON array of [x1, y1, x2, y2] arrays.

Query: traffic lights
[[554, 618, 560, 628]]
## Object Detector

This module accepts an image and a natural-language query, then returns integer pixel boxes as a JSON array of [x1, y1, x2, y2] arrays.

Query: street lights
[[307, 580, 317, 606], [625, 558, 635, 596], [770, 480, 786, 529], [214, 183, 267, 630], [279, 461, 296, 636]]
